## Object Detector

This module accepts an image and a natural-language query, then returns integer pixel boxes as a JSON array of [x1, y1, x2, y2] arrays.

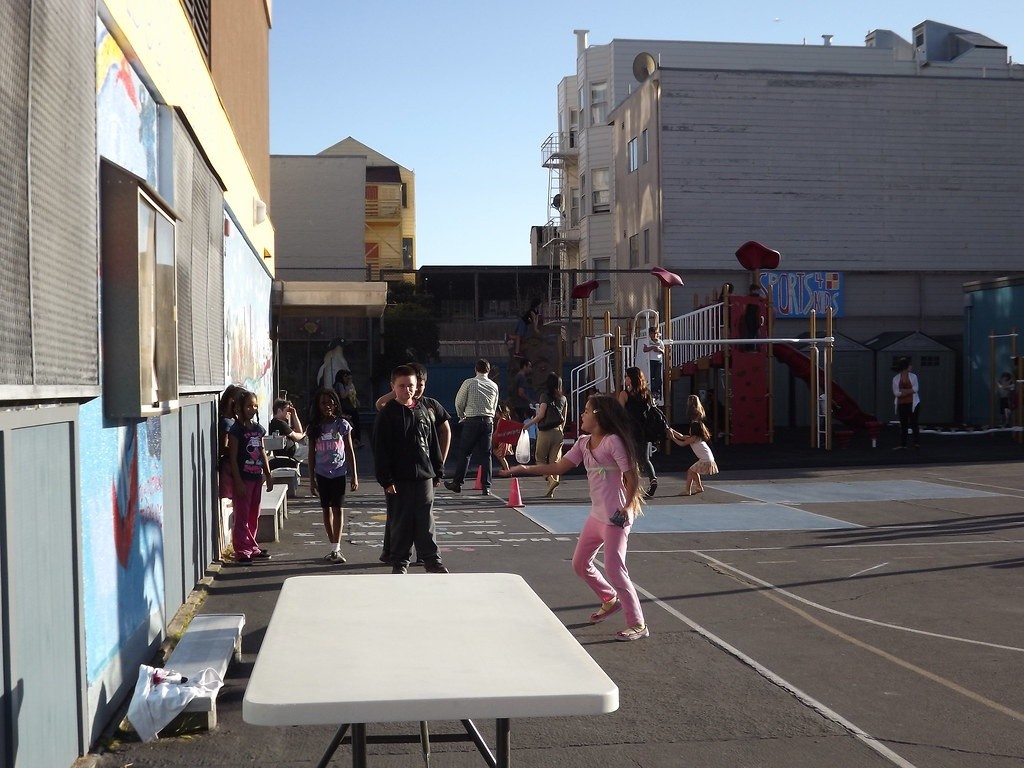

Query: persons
[[498, 396, 648, 641], [643, 326, 665, 397], [997, 372, 1014, 424], [306, 389, 358, 563], [269, 399, 309, 463], [370, 363, 452, 574], [668, 395, 718, 495], [333, 369, 365, 450], [218, 385, 272, 565], [504, 297, 541, 358], [523, 372, 568, 498], [508, 359, 535, 421], [715, 282, 734, 339], [891, 359, 920, 449], [492, 399, 539, 470], [445, 359, 498, 495], [743, 284, 761, 352], [618, 367, 658, 496]]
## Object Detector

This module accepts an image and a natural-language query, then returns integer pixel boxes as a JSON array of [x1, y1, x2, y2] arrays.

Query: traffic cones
[[473, 465, 483, 490], [505, 477, 526, 507]]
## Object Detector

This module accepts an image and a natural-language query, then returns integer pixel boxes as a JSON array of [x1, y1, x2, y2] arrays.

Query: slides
[[772, 343, 882, 431]]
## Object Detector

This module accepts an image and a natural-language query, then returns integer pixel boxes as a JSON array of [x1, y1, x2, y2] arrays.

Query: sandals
[[679, 490, 691, 496], [646, 480, 657, 496], [691, 488, 704, 495]]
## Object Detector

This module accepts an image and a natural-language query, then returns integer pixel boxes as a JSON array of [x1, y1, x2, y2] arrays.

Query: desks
[[242, 574, 620, 768]]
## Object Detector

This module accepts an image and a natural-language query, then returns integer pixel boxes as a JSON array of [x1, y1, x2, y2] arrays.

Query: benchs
[[255, 463, 300, 542], [162, 613, 246, 730]]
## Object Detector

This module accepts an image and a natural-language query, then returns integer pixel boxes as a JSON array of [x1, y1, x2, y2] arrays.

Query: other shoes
[[234, 549, 271, 565], [331, 551, 346, 563], [324, 554, 331, 560], [483, 488, 490, 495], [392, 564, 407, 574], [444, 481, 462, 493], [416, 558, 425, 566], [591, 598, 624, 622], [427, 563, 448, 573], [615, 625, 650, 640], [379, 556, 393, 566], [544, 480, 559, 497]]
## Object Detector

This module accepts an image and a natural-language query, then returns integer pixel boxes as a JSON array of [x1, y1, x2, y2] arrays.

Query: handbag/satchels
[[516, 428, 531, 464], [646, 406, 669, 442], [537, 401, 564, 431]]
